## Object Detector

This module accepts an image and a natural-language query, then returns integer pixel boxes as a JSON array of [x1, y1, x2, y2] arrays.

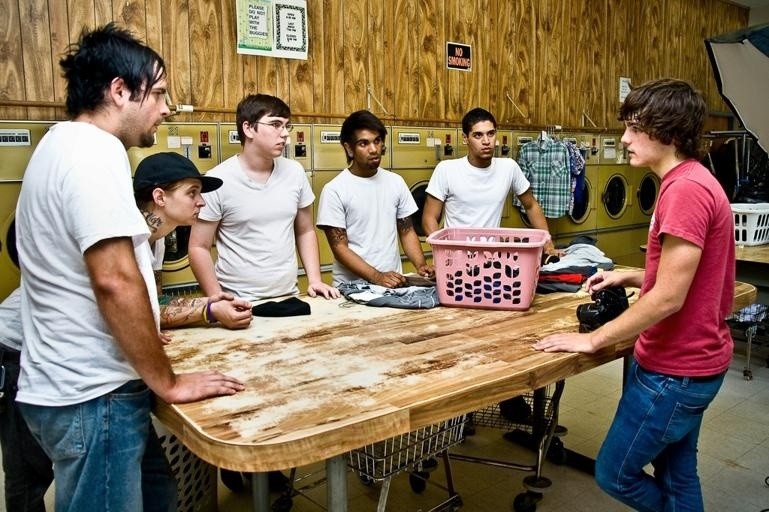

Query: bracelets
[[203, 302, 218, 325]]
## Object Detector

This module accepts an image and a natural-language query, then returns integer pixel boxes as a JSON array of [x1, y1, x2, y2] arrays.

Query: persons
[[0, 151, 252, 512], [529, 78, 738, 512], [13, 22, 245, 511], [422, 107, 568, 436], [315, 109, 436, 293], [188, 92, 342, 496]]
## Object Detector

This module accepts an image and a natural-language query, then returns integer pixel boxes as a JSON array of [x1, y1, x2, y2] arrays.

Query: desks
[[146, 255, 757, 511], [640, 240, 769, 367]]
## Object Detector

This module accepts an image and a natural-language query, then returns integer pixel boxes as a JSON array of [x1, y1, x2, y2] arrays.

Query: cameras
[[576, 285, 635, 332]]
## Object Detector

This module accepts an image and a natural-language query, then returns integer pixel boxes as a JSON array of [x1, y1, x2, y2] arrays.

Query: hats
[[133, 152, 223, 193]]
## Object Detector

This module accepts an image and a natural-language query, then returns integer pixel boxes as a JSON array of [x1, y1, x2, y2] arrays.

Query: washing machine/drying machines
[[0, 119, 710, 305]]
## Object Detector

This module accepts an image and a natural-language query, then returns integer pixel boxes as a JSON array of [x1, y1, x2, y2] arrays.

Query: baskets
[[729, 202, 769, 246], [151, 412, 217, 512], [425, 227, 551, 311]]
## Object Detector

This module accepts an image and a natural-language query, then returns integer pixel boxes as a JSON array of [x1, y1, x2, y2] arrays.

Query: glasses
[[250, 121, 294, 132]]
